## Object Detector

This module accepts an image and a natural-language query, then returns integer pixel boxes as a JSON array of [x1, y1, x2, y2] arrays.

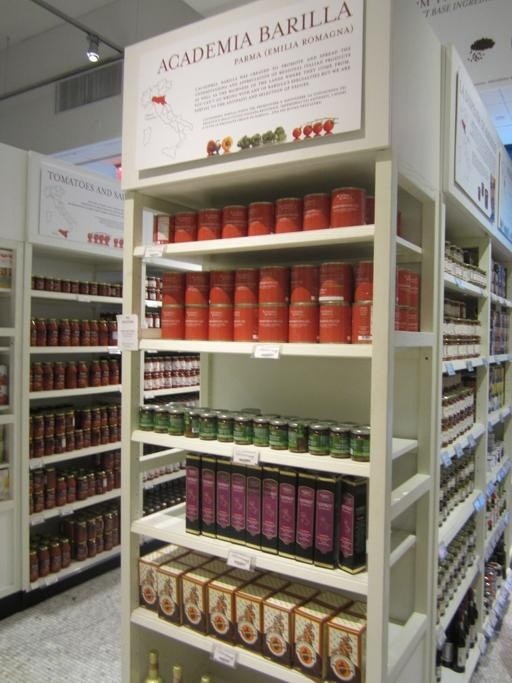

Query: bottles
[[435, 586, 481, 682], [143, 648, 210, 682], [485, 484, 508, 531]]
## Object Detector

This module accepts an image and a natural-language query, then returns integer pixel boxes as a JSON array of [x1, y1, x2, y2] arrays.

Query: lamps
[[87, 34, 100, 62]]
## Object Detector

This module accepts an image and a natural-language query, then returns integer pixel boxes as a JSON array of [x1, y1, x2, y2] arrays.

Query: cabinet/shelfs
[[0, 238, 198, 606], [120, 149, 440, 682], [436, 197, 512, 683]]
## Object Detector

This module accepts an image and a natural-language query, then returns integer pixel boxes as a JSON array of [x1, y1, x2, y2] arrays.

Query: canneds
[[153, 186, 401, 245], [436, 240, 486, 626], [30, 274, 122, 582], [161, 261, 421, 344], [138, 400, 374, 462]]
